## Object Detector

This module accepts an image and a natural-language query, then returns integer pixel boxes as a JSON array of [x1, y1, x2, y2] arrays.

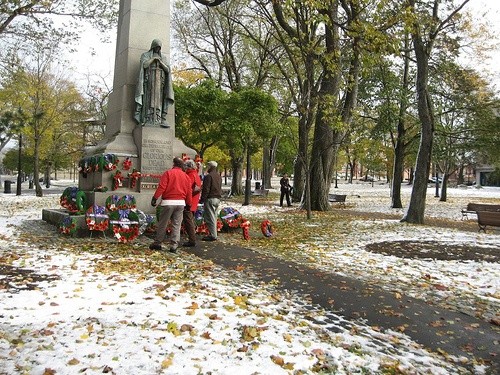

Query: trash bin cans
[[29, 180, 33, 189], [256, 182, 260, 189], [4, 180, 11, 193]]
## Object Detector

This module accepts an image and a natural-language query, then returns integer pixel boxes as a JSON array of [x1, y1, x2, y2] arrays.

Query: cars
[[359, 175, 377, 182], [428, 177, 442, 183]]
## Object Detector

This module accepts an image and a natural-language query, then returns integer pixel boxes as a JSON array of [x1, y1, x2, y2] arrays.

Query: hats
[[207, 161, 218, 168]]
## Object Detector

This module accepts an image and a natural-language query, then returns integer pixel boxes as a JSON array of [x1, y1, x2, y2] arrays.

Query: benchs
[[328, 194, 347, 209], [476, 210, 500, 234], [461, 203, 500, 221]]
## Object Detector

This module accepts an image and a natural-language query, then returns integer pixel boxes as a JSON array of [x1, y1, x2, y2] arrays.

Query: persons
[[134, 38, 175, 128], [280, 173, 293, 207], [198, 160, 223, 242], [184, 160, 202, 247], [149, 157, 193, 252]]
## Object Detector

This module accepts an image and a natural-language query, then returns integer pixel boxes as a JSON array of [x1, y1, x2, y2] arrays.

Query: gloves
[[151, 197, 157, 207]]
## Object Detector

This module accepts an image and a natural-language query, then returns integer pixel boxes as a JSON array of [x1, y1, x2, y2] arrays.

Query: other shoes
[[182, 242, 196, 247], [148, 244, 162, 250], [202, 236, 216, 241], [170, 248, 178, 253]]
